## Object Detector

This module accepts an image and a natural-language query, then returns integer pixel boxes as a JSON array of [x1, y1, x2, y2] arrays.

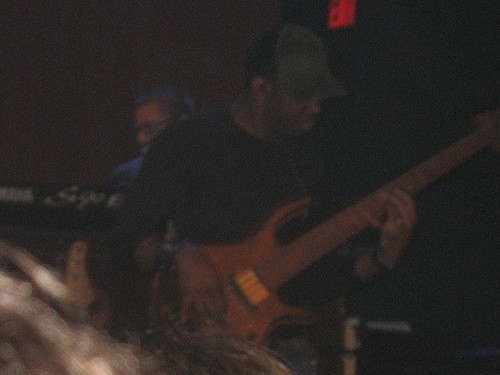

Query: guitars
[[150, 116, 500, 351]]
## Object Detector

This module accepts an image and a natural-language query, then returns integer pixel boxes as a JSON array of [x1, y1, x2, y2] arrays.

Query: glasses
[[131, 116, 174, 134]]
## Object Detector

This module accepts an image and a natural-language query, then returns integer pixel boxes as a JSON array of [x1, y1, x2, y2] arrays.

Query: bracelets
[[373, 254, 402, 273]]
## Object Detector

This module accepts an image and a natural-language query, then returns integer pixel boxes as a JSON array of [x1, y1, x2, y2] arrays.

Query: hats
[[246, 21, 351, 102]]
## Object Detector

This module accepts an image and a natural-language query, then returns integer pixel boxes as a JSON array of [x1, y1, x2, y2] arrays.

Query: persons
[[87, 20, 421, 374], [130, 81, 197, 144], [0, 238, 295, 375]]
